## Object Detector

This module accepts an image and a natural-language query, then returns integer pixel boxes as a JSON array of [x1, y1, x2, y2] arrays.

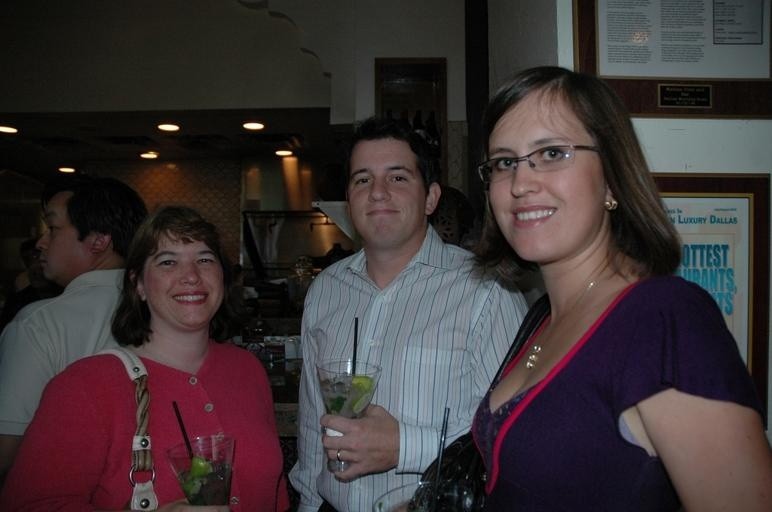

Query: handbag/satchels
[[407, 434, 521, 510]]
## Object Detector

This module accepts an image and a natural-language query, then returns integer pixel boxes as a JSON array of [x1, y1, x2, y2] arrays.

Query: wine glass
[[316, 358, 381, 473]]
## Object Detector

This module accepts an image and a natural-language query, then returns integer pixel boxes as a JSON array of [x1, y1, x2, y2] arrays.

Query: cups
[[164, 434, 236, 506]]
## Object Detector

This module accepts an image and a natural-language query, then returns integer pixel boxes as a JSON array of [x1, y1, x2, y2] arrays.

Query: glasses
[[477, 145, 600, 184]]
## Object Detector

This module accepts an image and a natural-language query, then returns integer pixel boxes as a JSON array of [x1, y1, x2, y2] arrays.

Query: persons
[[284, 111, 532, 511], [1, 238, 65, 332], [1, 172, 153, 472], [467, 65, 771, 511], [1, 206, 291, 511]]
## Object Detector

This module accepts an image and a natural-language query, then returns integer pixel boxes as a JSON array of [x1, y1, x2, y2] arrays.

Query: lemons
[[184, 456, 213, 493], [350, 377, 374, 412]]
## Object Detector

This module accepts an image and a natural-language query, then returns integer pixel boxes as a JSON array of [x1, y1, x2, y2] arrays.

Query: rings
[[337, 448, 343, 462]]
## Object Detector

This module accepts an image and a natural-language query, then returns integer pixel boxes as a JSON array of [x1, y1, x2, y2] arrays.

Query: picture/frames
[[655, 192, 755, 375]]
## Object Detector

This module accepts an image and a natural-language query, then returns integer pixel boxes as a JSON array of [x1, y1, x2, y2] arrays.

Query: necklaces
[[524, 272, 600, 372]]
